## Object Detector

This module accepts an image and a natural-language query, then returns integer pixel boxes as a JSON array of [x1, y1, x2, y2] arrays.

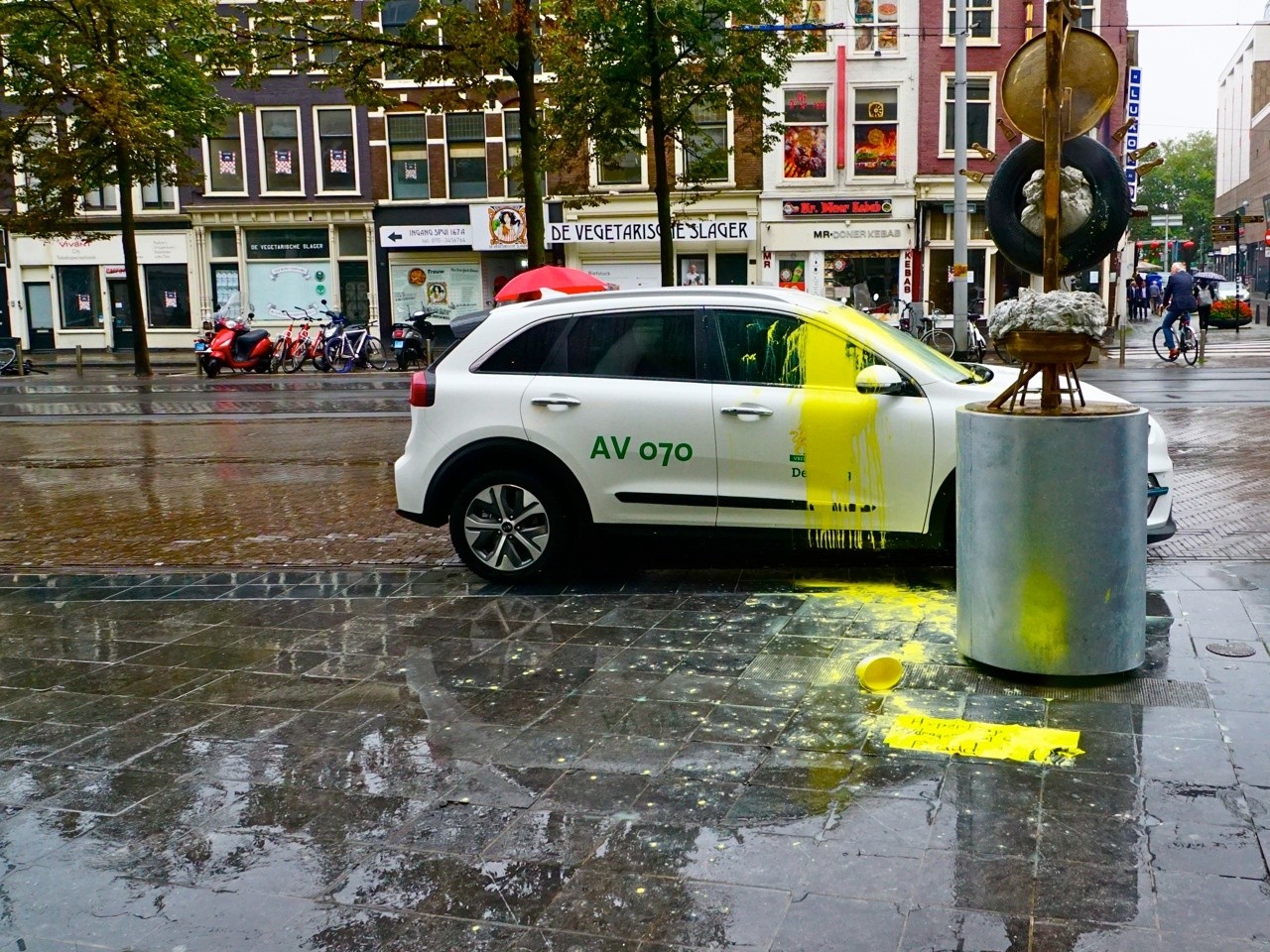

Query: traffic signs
[[379, 224, 471, 247]]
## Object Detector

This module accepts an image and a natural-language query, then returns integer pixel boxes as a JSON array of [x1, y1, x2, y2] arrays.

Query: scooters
[[192, 314, 273, 378], [391, 302, 438, 372]]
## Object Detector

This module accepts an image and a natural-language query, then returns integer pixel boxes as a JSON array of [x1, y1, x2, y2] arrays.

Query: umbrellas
[[495, 266, 608, 303], [1194, 272, 1228, 281]]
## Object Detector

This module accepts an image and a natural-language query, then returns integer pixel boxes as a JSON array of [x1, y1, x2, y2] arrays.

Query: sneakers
[[1186, 329, 1192, 340], [1169, 349, 1179, 357]]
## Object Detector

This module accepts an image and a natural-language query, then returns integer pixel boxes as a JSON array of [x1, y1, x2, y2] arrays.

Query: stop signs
[[1265, 229, 1270, 247]]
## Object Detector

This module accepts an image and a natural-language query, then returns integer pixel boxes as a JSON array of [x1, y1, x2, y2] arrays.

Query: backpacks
[[1197, 284, 1212, 306]]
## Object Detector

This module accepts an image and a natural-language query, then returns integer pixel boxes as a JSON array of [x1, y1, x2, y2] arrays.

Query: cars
[[1213, 281, 1251, 307]]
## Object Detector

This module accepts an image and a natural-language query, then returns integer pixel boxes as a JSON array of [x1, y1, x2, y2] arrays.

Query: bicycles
[[967, 298, 987, 364], [0, 347, 33, 376], [1152, 302, 1198, 366], [273, 299, 388, 374], [893, 298, 956, 359]]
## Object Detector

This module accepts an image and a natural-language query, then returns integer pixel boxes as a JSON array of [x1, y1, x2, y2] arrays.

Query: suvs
[[392, 285, 1178, 587]]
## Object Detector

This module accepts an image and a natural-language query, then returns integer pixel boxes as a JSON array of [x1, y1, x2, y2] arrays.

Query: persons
[[516, 291, 542, 303], [1148, 280, 1160, 313], [1157, 261, 1199, 358], [1127, 281, 1140, 323], [1139, 281, 1150, 321], [683, 264, 703, 286], [1193, 278, 1216, 335]]
